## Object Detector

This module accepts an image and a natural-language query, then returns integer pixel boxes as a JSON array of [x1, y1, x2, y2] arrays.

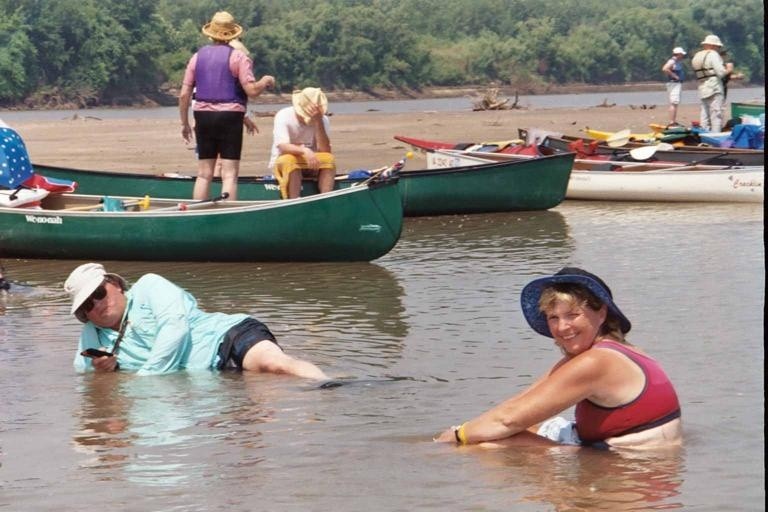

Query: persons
[[186, 32, 259, 151], [690, 35, 733, 133], [178, 10, 275, 201], [266, 85, 337, 200], [718, 47, 744, 111], [434, 263, 684, 452], [62, 262, 331, 383], [661, 45, 687, 126]]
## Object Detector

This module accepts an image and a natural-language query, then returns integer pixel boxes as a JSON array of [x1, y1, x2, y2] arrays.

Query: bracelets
[[454, 429, 461, 444], [460, 421, 469, 445]]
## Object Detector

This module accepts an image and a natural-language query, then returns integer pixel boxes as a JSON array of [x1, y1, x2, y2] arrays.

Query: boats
[[0, 162, 404, 263], [0, 257, 405, 384], [396, 210, 574, 265], [161, 149, 577, 215]]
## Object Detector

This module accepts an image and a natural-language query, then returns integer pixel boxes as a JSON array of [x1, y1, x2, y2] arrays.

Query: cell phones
[[85, 347, 113, 357]]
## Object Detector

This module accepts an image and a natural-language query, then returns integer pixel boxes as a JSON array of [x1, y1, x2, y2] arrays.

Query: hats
[[700, 34, 724, 47], [673, 46, 687, 55], [520, 265, 633, 337], [201, 10, 242, 42], [63, 260, 107, 313], [291, 86, 328, 126]]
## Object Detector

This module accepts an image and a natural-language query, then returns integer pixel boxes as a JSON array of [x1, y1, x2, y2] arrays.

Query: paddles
[[613, 146, 658, 161], [584, 129, 629, 147]]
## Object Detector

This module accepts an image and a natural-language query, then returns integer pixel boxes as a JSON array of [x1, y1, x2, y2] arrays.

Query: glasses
[[83, 285, 107, 311]]
